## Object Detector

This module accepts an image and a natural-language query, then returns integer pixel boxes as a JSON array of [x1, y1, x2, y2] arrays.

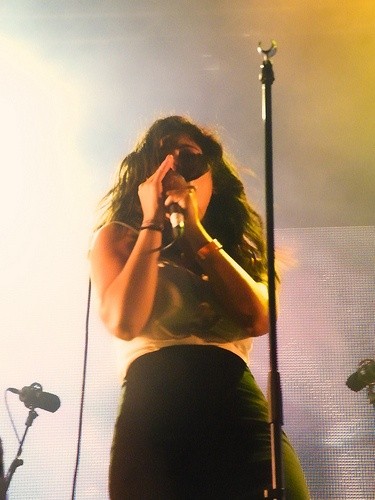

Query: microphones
[[346, 359, 375, 391], [7, 386, 60, 413], [168, 203, 186, 241]]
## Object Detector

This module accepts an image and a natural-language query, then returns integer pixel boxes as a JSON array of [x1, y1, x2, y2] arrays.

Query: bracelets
[[136, 220, 165, 232], [189, 239, 224, 264]]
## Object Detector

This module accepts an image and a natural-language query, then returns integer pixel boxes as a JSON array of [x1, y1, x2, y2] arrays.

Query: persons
[[84, 113, 312, 500]]
